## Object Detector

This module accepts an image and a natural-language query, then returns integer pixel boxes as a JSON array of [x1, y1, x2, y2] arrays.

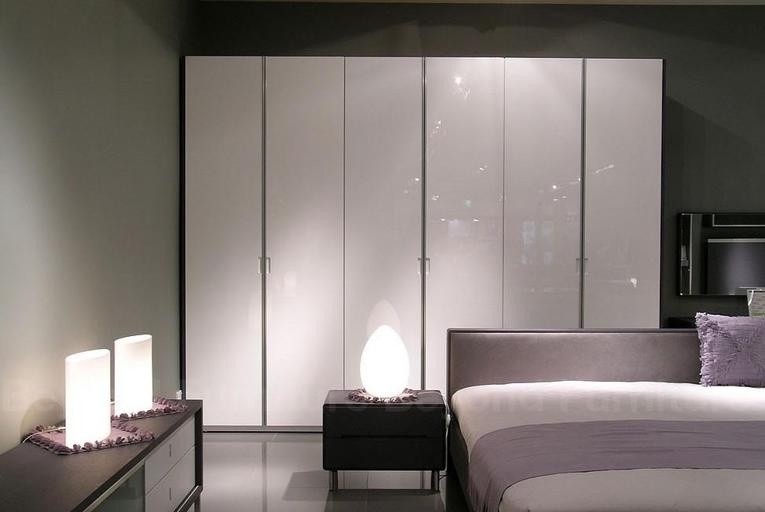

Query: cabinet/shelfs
[[0, 397, 206, 511], [498, 53, 671, 334], [177, 53, 345, 435], [340, 51, 504, 407]]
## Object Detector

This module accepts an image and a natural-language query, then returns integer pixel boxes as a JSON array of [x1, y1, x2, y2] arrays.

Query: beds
[[440, 325, 765, 512]]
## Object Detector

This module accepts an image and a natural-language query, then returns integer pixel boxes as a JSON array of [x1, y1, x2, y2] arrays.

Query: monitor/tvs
[[675, 211, 765, 297]]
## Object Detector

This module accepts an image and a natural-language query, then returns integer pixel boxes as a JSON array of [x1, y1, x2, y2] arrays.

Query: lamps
[[113, 332, 155, 418], [358, 320, 412, 401], [62, 348, 112, 451]]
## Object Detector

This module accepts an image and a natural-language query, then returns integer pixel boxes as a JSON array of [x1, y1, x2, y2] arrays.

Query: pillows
[[693, 311, 765, 390]]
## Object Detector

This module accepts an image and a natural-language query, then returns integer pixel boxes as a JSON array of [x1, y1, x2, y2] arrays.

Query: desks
[[666, 313, 750, 330], [321, 388, 447, 493]]
